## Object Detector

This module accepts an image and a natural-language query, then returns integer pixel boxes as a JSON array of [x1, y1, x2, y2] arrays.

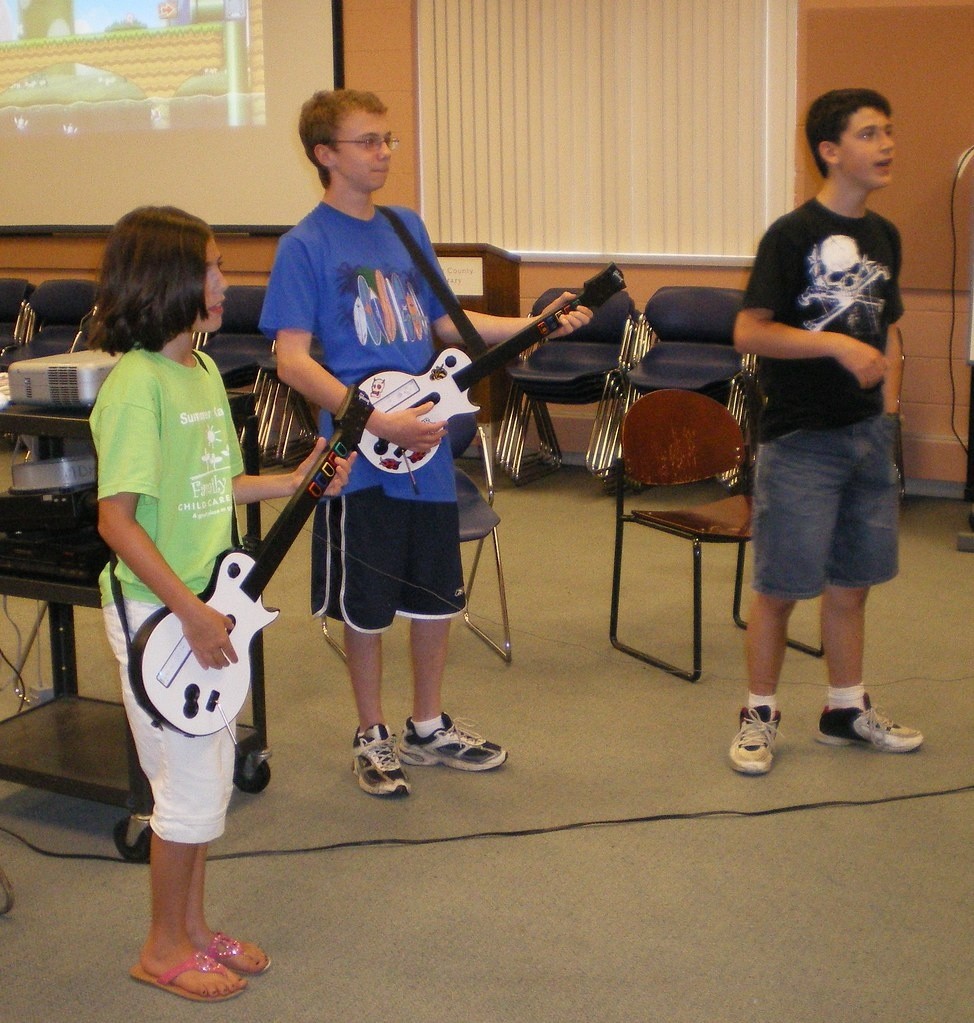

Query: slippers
[[210, 929, 272, 978], [129, 954, 244, 1001]]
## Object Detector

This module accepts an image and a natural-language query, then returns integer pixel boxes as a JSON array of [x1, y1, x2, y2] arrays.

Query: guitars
[[129, 383, 375, 737], [355, 260, 627, 478]]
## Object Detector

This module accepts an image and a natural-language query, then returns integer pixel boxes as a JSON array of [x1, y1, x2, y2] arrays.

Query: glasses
[[316, 135, 401, 152]]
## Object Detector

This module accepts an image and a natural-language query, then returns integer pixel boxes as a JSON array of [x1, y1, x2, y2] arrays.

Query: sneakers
[[817, 693, 925, 751], [351, 725, 412, 797], [729, 707, 784, 775], [396, 711, 510, 773]]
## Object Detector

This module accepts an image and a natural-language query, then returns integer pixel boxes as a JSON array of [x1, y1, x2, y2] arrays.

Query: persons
[[88, 199, 359, 1001], [255, 88, 509, 797], [723, 88, 931, 777]]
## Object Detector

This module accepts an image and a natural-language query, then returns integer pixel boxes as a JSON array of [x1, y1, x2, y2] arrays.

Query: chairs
[[0, 271, 836, 689]]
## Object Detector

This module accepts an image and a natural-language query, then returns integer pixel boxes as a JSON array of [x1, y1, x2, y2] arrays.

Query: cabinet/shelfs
[[0, 389, 274, 857]]
